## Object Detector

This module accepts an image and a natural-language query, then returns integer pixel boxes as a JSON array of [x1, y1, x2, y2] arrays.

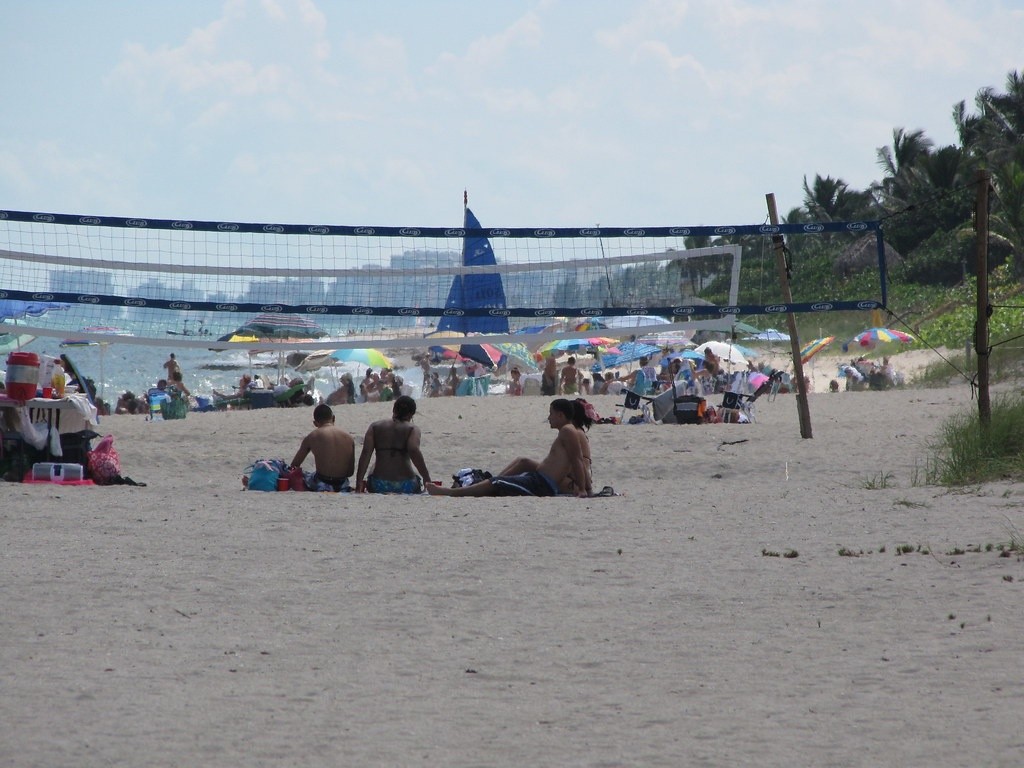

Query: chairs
[[146, 386, 177, 420], [215, 369, 786, 425]]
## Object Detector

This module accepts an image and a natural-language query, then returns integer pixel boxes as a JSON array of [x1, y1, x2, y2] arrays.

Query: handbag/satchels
[[248, 458, 306, 492]]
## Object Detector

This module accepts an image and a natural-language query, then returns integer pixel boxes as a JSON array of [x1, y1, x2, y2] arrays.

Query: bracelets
[[586, 488, 592, 490]]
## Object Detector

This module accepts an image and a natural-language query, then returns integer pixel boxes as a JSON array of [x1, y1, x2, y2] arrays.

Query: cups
[[278, 478, 289, 490], [432, 481, 443, 486], [43, 388, 52, 398]]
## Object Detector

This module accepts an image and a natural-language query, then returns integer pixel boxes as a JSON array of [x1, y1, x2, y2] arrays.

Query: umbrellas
[[873, 309, 883, 328], [0, 331, 38, 357], [1, 298, 70, 354], [427, 337, 794, 380], [840, 328, 920, 353], [208, 311, 332, 386], [286, 345, 392, 389], [740, 328, 792, 350], [604, 314, 671, 372], [786, 336, 837, 374], [711, 318, 762, 342], [58, 326, 139, 397], [512, 320, 609, 332]]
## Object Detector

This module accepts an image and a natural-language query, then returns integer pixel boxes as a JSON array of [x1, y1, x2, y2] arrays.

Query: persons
[[85, 352, 191, 415], [355, 396, 431, 494], [541, 355, 681, 409], [211, 374, 314, 406], [503, 367, 524, 395], [414, 351, 479, 398], [764, 369, 781, 402], [844, 355, 898, 386], [425, 398, 595, 497], [689, 347, 766, 394], [242, 404, 355, 492], [336, 365, 404, 403]]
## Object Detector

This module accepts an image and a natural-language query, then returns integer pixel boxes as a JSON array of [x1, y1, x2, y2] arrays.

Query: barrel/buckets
[[5, 352, 41, 400]]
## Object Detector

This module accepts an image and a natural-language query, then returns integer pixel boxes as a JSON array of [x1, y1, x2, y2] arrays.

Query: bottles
[[52, 358, 65, 399], [615, 410, 621, 424]]
